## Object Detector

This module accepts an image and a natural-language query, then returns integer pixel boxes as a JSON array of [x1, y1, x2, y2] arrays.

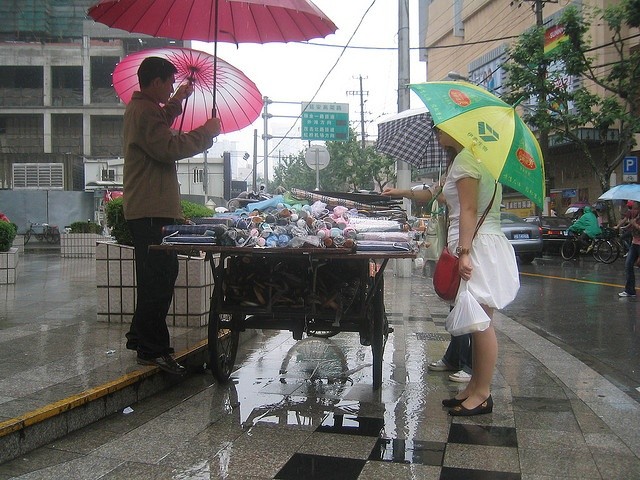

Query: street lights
[[447, 71, 552, 216]]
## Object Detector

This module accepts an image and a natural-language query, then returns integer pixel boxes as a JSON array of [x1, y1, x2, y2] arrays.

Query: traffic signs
[[301, 101, 349, 142]]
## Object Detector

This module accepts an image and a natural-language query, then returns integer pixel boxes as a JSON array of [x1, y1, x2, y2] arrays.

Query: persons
[[618, 202, 640, 297], [568, 205, 603, 254], [381, 118, 480, 386], [612, 200, 640, 256], [120, 56, 227, 380], [431, 120, 522, 417]]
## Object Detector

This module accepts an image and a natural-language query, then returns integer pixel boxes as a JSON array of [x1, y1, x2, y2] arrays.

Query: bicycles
[[23, 219, 60, 245], [560, 222, 630, 264]]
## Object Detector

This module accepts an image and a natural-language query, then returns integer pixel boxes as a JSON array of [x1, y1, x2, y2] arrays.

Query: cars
[[500, 212, 543, 263], [523, 216, 572, 251]]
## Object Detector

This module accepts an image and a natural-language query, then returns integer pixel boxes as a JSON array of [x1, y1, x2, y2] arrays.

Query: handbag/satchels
[[432, 180, 497, 303]]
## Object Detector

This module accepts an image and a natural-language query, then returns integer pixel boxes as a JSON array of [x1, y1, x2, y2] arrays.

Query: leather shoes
[[126, 342, 174, 353], [138, 348, 188, 378]]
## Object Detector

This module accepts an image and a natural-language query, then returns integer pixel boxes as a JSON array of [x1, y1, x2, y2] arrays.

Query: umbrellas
[[350, 216, 431, 252], [597, 183, 640, 202], [110, 46, 266, 138], [161, 223, 227, 237], [374, 105, 454, 188], [163, 238, 218, 243], [293, 216, 350, 249], [294, 186, 409, 216], [187, 217, 235, 228], [85, 0, 340, 119], [226, 181, 293, 247]]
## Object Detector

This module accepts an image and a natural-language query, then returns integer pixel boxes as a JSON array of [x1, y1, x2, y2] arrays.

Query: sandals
[[443, 397, 467, 407], [447, 394, 496, 416]]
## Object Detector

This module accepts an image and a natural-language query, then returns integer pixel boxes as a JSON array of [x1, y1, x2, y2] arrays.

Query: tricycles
[[202, 185, 394, 389]]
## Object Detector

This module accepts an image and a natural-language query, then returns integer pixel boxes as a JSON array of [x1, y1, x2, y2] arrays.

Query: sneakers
[[619, 292, 636, 298], [585, 245, 593, 255], [427, 360, 447, 371], [449, 370, 472, 382]]
